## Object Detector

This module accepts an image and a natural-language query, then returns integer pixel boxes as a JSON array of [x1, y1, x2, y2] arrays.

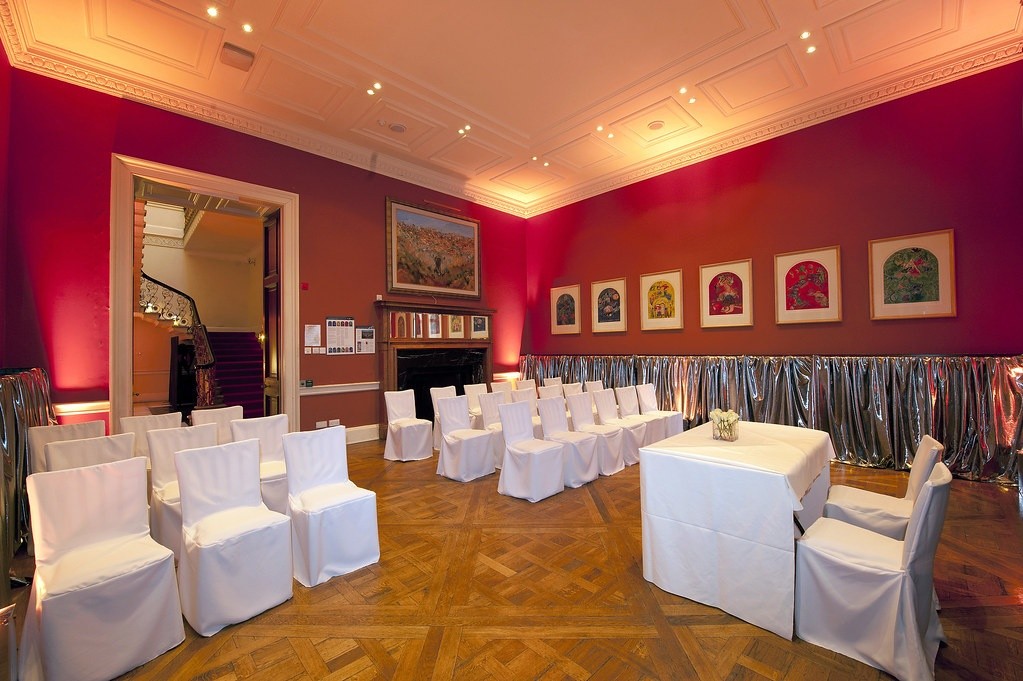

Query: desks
[[637, 418, 830, 638]]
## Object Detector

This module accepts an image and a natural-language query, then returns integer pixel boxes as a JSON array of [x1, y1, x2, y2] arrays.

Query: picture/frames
[[774, 245, 844, 324], [869, 228, 957, 322], [640, 268, 684, 331], [385, 195, 482, 301], [550, 283, 582, 335], [591, 276, 628, 333], [699, 258, 755, 329]]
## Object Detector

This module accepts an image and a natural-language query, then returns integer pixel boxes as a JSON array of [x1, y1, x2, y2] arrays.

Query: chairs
[[826, 433, 945, 542], [18, 455, 186, 681], [795, 460, 953, 681], [172, 438, 292, 638], [384, 376, 685, 502], [280, 425, 381, 589], [26, 404, 289, 564]]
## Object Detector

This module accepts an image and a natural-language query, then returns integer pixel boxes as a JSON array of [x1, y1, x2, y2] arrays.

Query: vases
[[711, 420, 740, 441]]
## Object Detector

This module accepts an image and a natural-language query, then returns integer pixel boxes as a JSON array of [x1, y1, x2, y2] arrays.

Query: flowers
[[709, 408, 739, 438]]
